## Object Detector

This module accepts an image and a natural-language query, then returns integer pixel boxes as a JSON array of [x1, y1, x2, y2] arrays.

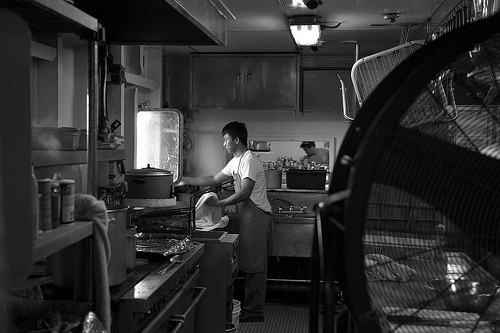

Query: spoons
[[156, 255, 184, 275]]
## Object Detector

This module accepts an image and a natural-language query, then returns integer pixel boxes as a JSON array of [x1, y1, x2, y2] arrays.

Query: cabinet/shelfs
[[189, 53, 304, 112]]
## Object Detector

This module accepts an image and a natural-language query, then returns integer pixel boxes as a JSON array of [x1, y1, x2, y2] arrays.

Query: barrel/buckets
[[104, 205, 133, 286], [126, 224, 138, 272], [286, 168, 327, 190], [264, 169, 282, 189]]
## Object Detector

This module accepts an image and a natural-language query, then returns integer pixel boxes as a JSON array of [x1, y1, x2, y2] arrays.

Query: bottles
[[37, 179, 52, 231], [60, 179, 76, 224], [49, 182, 61, 229]]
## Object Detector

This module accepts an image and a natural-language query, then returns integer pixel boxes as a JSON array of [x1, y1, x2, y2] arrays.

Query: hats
[[300, 141, 315, 147]]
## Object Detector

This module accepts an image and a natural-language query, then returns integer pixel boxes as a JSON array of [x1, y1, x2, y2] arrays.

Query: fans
[[324, 11, 500, 332]]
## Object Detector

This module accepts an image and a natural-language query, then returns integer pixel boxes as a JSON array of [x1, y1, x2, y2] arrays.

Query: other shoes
[[239, 310, 265, 322]]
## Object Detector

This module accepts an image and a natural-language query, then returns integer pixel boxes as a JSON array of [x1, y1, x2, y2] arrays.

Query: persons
[[300, 141, 329, 166], [179, 121, 272, 321]]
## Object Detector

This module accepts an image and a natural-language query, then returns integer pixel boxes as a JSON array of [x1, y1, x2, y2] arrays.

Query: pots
[[124, 163, 174, 199]]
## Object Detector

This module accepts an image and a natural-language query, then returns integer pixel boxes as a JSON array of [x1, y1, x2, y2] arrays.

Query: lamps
[[288, 15, 322, 48]]
